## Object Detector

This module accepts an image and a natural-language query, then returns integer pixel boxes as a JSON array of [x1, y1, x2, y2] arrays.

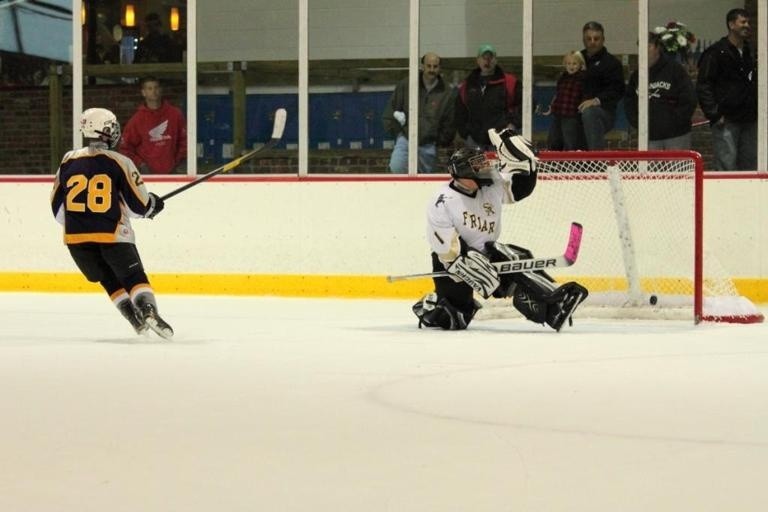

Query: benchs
[[533, 129, 629, 150]]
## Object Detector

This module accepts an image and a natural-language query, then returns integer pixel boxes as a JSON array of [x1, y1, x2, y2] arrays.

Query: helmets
[[449, 145, 501, 189], [80, 108, 122, 148]]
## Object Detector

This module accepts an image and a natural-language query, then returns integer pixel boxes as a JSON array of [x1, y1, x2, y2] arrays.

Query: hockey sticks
[[160, 107, 288, 201], [605, 158, 657, 306], [388, 222, 584, 282]]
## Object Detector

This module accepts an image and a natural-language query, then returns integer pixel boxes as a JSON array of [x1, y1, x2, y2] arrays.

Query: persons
[[412, 114, 590, 332], [697, 8, 756, 172], [439, 43, 509, 151], [626, 33, 698, 152], [120, 74, 185, 176], [379, 50, 452, 173], [50, 108, 176, 339], [541, 49, 591, 151], [0, 11, 182, 90], [570, 20, 625, 152]]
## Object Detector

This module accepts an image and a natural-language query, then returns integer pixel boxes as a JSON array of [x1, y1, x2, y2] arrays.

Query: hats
[[478, 44, 496, 57]]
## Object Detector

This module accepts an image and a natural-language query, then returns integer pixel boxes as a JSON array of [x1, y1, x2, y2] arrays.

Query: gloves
[[143, 191, 164, 219]]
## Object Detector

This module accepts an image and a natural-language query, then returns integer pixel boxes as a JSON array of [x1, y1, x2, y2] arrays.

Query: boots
[[134, 292, 174, 337], [118, 298, 146, 333]]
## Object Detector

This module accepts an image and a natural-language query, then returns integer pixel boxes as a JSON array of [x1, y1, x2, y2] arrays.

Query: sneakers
[[548, 290, 583, 332]]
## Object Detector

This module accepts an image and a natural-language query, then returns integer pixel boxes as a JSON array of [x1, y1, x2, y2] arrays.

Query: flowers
[[649, 21, 696, 63]]
